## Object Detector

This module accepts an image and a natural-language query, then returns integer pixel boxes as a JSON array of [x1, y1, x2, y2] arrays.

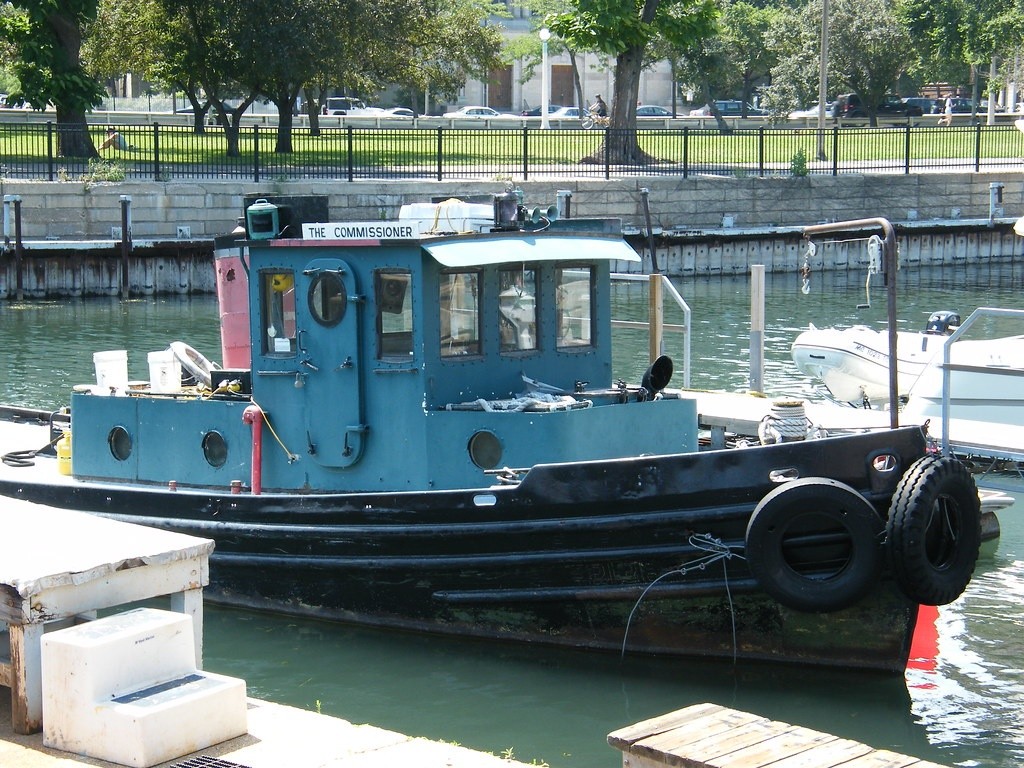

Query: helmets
[[596, 94, 600, 98]]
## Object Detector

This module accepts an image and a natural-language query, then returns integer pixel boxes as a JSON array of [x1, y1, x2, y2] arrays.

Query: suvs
[[829, 93, 923, 127]]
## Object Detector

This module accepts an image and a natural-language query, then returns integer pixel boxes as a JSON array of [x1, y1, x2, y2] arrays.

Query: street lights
[[539, 29, 551, 129]]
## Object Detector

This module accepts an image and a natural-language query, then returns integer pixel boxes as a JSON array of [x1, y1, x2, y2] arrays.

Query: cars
[[898, 96, 1006, 113], [689, 99, 771, 116], [522, 104, 561, 117], [636, 105, 688, 117], [300, 96, 441, 118], [788, 103, 831, 117], [548, 106, 592, 118], [176, 100, 239, 115], [442, 106, 521, 119], [0, 91, 39, 110]]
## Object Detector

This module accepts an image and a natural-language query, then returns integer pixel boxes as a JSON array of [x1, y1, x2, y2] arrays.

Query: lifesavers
[[885, 451, 982, 607], [170, 341, 217, 388], [744, 476, 888, 614]]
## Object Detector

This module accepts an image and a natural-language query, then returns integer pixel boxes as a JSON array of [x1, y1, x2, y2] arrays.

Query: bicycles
[[581, 109, 611, 130]]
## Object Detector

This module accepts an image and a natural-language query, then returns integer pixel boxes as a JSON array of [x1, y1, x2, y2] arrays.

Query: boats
[[615, 298, 1024, 465], [0, 184, 1002, 679]]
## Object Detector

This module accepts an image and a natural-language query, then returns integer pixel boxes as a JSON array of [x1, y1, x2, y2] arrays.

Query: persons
[[590, 94, 606, 124], [637, 102, 641, 106], [96, 128, 127, 150], [938, 93, 956, 126]]
